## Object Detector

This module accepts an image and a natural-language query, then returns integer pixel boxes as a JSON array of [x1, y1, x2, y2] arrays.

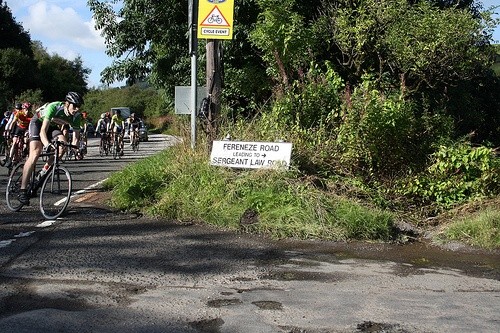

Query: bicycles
[[94, 130, 141, 160], [5, 137, 79, 220], [0, 131, 88, 176]]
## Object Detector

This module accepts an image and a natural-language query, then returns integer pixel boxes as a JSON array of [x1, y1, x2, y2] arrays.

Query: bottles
[[40, 162, 50, 176]]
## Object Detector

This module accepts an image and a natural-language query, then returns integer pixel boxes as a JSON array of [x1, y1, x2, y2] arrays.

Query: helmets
[[66, 91, 84, 104], [14, 102, 33, 109], [131, 113, 137, 117], [4, 111, 12, 117], [114, 109, 121, 113], [101, 112, 111, 118]]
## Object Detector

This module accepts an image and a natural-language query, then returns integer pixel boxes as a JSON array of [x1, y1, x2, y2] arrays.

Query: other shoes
[[34, 171, 46, 187], [6, 157, 12, 167], [16, 189, 30, 204]]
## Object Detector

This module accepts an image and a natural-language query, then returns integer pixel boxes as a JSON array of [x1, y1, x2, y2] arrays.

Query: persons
[[17, 92, 86, 205], [94, 112, 114, 154], [128, 113, 140, 148], [0, 102, 34, 166], [110, 109, 125, 152]]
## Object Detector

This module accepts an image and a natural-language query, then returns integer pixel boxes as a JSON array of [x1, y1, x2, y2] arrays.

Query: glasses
[[69, 102, 81, 108]]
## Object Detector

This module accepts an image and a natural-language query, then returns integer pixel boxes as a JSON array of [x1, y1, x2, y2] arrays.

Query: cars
[[123, 120, 148, 142]]
